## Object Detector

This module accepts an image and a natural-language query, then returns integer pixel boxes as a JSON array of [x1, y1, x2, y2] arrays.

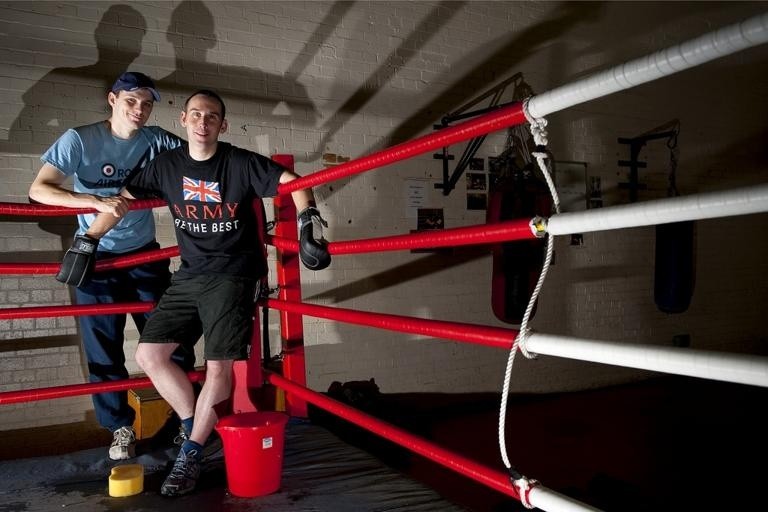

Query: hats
[[112, 72, 160, 101]]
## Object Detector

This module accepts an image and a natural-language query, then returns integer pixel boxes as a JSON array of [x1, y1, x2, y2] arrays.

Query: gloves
[[55, 235, 99, 288], [297, 207, 331, 270]]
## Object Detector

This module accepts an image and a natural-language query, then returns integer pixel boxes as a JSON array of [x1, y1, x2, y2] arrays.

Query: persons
[[418, 210, 435, 229], [53, 90, 333, 496], [29, 70, 224, 461]]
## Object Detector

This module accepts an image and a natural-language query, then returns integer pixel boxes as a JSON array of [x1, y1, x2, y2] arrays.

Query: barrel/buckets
[[214, 412, 288, 498]]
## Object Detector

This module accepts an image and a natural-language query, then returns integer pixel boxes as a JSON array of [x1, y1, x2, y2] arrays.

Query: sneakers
[[109, 425, 225, 498]]
